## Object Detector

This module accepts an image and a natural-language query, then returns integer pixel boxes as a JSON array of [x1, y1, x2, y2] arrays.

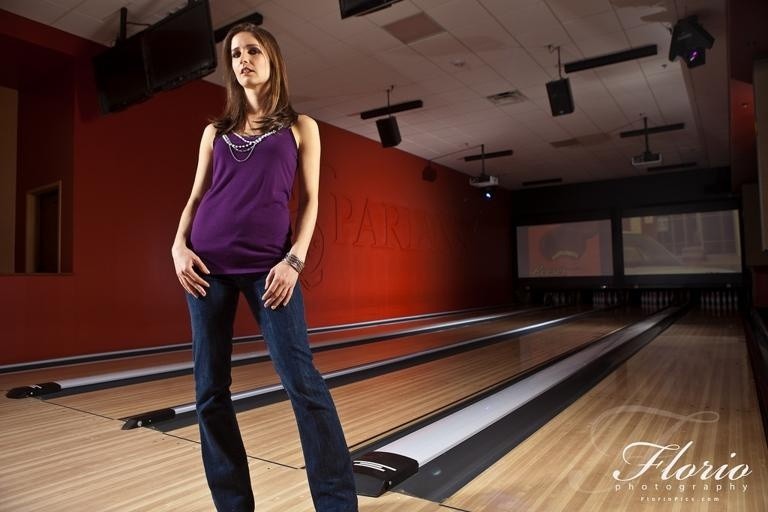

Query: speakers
[[376, 116, 401, 148], [546, 77, 575, 117]]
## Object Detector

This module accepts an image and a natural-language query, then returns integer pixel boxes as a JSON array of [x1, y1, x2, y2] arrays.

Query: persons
[[170, 25, 358, 511]]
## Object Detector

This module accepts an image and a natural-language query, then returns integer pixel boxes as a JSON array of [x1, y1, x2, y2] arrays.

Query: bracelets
[[283, 254, 305, 273]]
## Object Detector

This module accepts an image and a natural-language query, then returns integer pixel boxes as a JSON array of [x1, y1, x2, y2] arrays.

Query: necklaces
[[221, 123, 285, 163]]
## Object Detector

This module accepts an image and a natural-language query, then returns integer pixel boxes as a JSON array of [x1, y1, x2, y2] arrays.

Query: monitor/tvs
[[339, 0, 402, 19], [93, 0, 218, 114]]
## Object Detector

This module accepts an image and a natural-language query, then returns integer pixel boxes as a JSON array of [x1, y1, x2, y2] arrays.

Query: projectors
[[468, 175, 499, 188], [631, 152, 663, 169]]
